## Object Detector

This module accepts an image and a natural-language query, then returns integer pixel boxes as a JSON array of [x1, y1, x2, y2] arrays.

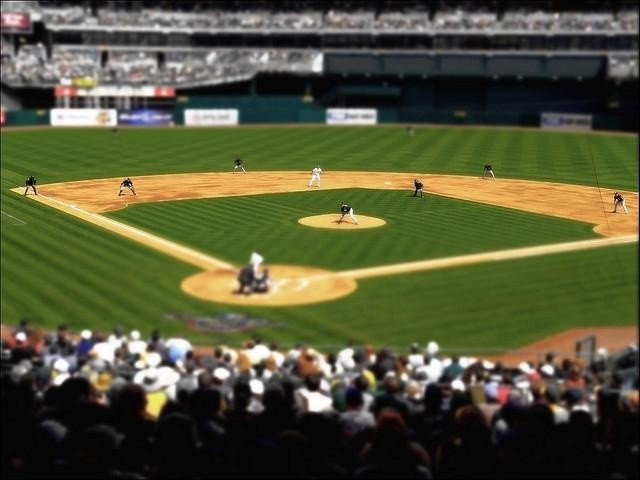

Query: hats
[[134, 367, 179, 393]]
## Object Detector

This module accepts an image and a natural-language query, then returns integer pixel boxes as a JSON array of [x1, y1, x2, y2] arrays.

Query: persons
[[337, 202, 360, 226], [308, 164, 323, 188], [233, 157, 248, 174], [236, 264, 258, 295], [413, 178, 426, 199], [0, 318, 638, 479], [24, 176, 38, 196], [611, 191, 629, 215], [253, 270, 272, 293], [118, 177, 137, 198], [248, 249, 266, 281], [482, 162, 496, 182]]
